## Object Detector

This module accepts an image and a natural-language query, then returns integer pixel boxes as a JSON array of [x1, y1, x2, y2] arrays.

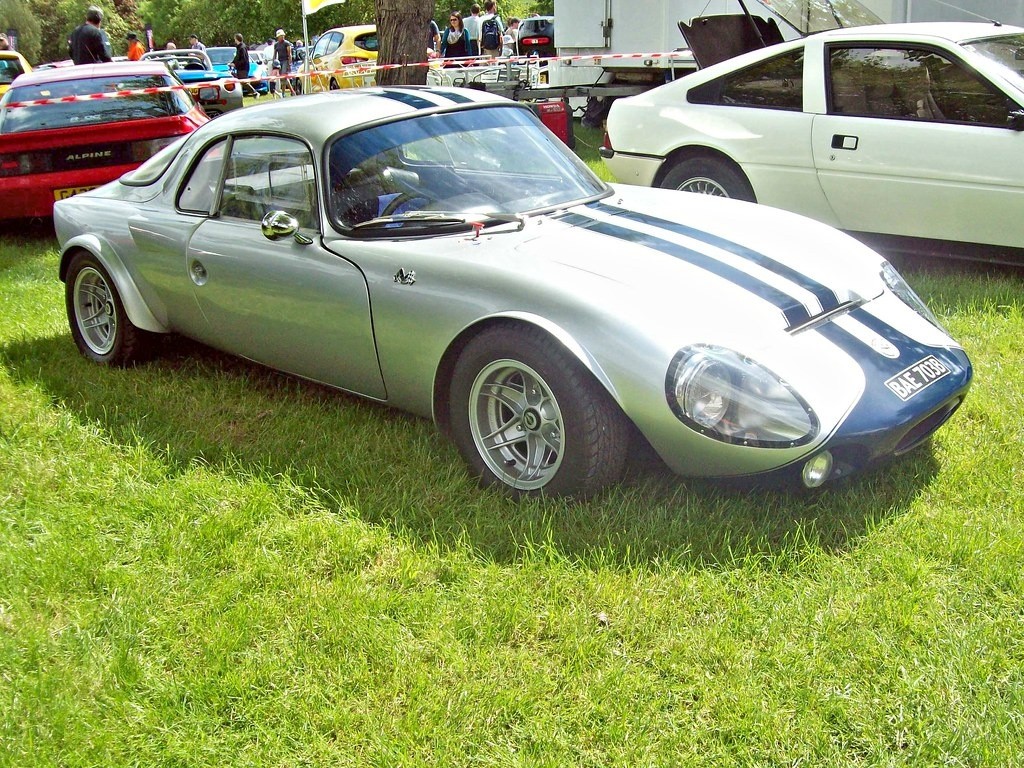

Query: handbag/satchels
[[585, 95, 608, 125]]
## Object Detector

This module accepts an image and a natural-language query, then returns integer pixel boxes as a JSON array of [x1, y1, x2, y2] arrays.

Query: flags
[[303, 0, 345, 16]]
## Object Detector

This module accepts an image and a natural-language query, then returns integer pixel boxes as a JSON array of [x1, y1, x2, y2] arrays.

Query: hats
[[503, 35, 514, 44], [276, 29, 286, 37], [0, 33, 7, 40], [272, 59, 281, 67], [187, 34, 198, 39], [126, 33, 137, 40]]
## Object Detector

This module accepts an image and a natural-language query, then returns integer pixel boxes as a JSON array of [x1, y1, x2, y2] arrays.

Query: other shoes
[[253, 92, 260, 100]]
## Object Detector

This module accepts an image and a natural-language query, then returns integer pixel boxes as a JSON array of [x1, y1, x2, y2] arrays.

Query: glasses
[[450, 19, 457, 21]]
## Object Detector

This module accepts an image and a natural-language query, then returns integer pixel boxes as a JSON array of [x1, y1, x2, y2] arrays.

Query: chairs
[[183, 62, 206, 70], [831, 64, 948, 121]]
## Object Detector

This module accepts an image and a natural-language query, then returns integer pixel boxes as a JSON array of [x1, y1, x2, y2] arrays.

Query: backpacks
[[482, 15, 500, 50]]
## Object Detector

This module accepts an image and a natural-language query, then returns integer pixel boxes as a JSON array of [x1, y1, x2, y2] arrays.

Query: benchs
[[220, 167, 420, 231]]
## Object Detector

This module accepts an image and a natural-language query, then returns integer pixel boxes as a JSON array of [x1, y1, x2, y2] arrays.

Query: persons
[[263, 36, 304, 73], [227, 33, 260, 100], [164, 42, 178, 63], [269, 59, 282, 100], [126, 32, 146, 61], [436, 11, 473, 69], [502, 16, 520, 57], [478, 0, 505, 58], [187, 34, 207, 63], [70, 4, 115, 66], [0, 32, 15, 52], [273, 30, 296, 98], [462, 3, 481, 67], [426, 20, 441, 59]]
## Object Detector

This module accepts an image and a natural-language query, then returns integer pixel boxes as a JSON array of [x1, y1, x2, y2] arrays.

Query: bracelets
[[436, 49, 440, 52]]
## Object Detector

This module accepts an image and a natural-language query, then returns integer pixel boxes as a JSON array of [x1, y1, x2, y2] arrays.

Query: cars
[[1, 50, 33, 99], [138, 50, 243, 120], [599, 1, 1024, 269], [292, 25, 441, 95], [52, 84, 974, 505], [207, 47, 270, 96], [0, 60, 220, 232]]
